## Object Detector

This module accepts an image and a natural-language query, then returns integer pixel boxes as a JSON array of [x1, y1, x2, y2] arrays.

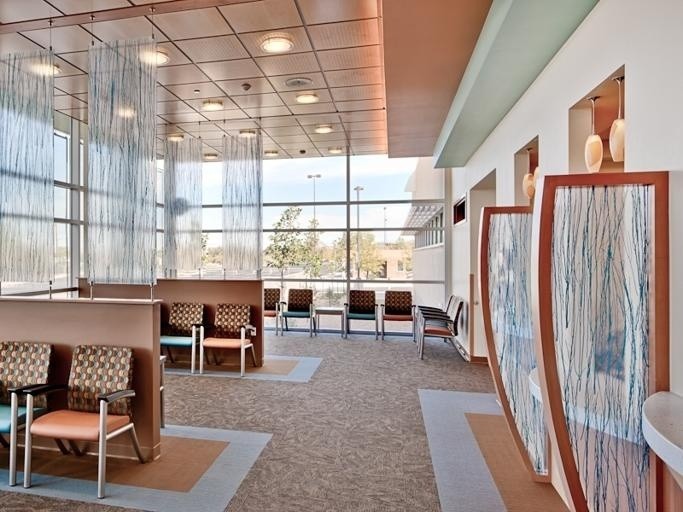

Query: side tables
[[314, 306, 344, 338]]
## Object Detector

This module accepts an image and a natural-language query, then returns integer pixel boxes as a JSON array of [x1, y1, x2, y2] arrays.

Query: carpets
[[417, 388, 572, 512], [164, 354, 325, 384], [0, 424, 276, 512]]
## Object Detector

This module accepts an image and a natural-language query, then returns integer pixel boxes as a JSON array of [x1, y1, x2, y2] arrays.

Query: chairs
[[416, 293, 465, 360], [198, 303, 258, 378], [280, 289, 317, 337], [160, 302, 211, 375], [0, 341, 69, 486], [380, 290, 417, 342], [343, 290, 379, 340], [159, 356, 167, 430], [22, 344, 146, 499], [264, 287, 280, 334]]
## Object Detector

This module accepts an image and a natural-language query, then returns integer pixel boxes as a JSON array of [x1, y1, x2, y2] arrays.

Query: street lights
[[306, 175, 322, 253], [353, 185, 363, 267]]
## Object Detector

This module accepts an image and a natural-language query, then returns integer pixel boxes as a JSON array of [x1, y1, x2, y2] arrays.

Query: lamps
[[609, 75, 625, 162], [583, 95, 604, 173], [522, 147, 535, 199], [533, 165, 539, 191]]
